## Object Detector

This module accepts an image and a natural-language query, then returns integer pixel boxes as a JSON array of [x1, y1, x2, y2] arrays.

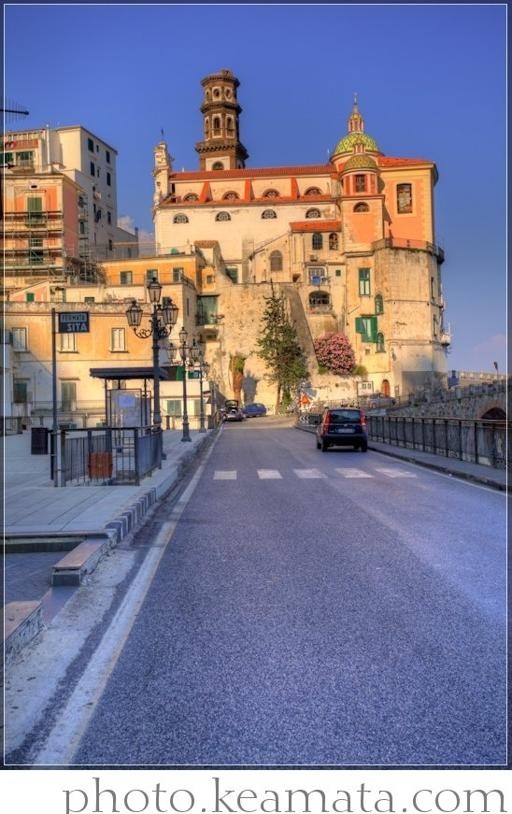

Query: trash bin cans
[[31, 427, 49, 455]]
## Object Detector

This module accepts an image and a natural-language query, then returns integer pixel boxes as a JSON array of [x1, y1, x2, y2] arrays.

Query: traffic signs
[[58, 312, 89, 334]]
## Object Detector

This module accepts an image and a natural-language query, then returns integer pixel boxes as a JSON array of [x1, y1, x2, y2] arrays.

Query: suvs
[[316, 406, 369, 452]]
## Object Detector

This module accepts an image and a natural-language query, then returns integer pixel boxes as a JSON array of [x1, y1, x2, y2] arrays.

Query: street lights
[[201, 362, 218, 430], [168, 326, 199, 441], [126, 276, 179, 464]]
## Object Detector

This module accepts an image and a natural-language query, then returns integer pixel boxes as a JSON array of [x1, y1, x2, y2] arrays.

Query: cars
[[220, 398, 266, 422]]
